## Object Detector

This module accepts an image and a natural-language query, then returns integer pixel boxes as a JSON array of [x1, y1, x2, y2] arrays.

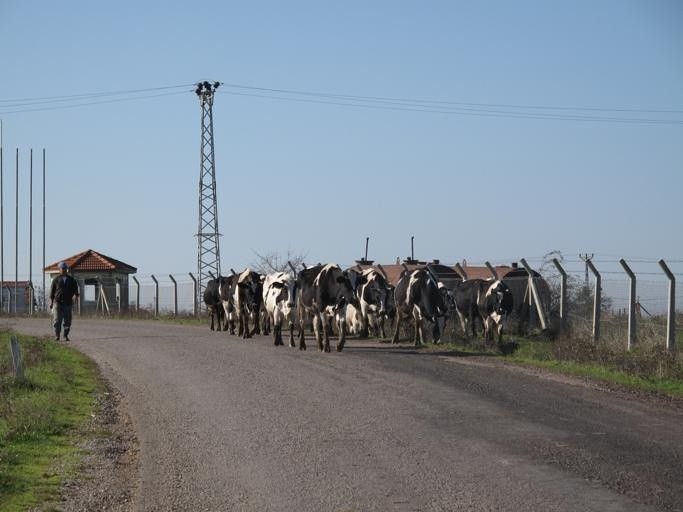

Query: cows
[[203, 263, 452, 353], [450, 278, 515, 350]]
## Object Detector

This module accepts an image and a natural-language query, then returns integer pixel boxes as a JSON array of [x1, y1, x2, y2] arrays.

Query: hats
[[58, 262, 68, 270]]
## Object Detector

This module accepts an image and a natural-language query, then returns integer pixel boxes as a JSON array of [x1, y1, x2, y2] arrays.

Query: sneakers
[[54, 336, 70, 342]]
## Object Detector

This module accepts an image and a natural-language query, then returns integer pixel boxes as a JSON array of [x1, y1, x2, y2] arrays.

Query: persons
[[49, 261, 80, 341]]
